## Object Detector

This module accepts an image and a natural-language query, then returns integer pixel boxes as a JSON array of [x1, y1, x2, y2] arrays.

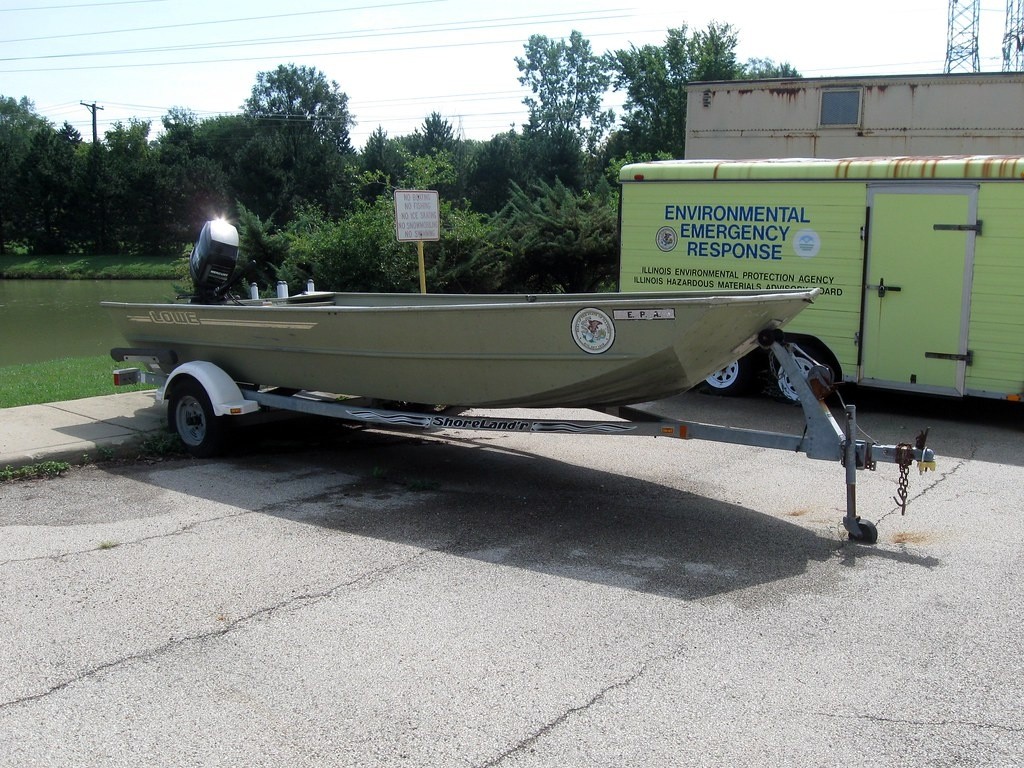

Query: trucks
[[617, 157, 1024, 411]]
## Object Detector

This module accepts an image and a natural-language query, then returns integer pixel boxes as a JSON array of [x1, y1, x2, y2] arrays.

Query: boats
[[99, 216, 936, 545]]
